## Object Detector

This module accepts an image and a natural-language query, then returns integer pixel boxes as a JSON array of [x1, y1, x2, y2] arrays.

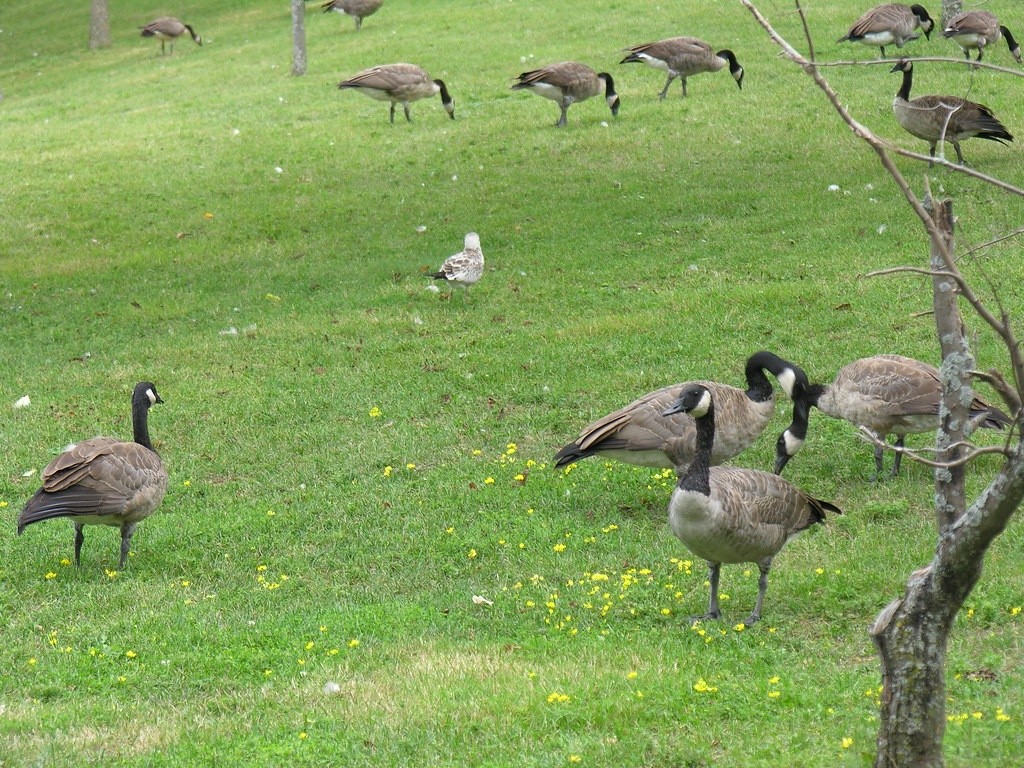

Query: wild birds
[[890, 56, 1015, 169], [550, 351, 811, 476], [663, 384, 842, 627], [321, 0, 383, 30], [141, 17, 203, 57], [939, 10, 1023, 71], [618, 35, 745, 101], [17, 382, 167, 573], [508, 62, 621, 125], [836, 4, 934, 58], [810, 354, 1015, 481], [336, 63, 455, 125], [422, 232, 484, 303]]
